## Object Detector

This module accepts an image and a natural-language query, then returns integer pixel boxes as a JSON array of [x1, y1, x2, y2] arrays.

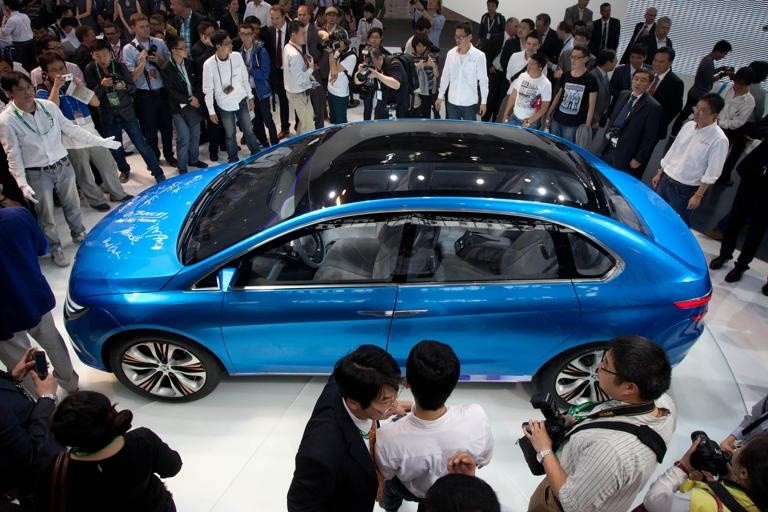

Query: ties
[[648, 74, 660, 96], [601, 21, 609, 50], [635, 24, 648, 47], [367, 419, 385, 503], [614, 95, 637, 129], [276, 28, 283, 66]]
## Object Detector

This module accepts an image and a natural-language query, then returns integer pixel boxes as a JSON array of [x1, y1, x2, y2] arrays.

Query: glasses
[[692, 105, 710, 114], [370, 393, 399, 417], [597, 362, 618, 378], [240, 31, 253, 37], [104, 31, 118, 37], [47, 44, 66, 51]]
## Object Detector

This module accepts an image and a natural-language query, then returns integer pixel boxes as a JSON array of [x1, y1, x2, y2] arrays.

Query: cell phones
[[31, 349, 49, 380]]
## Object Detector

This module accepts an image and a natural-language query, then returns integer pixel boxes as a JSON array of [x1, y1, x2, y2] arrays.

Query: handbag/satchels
[[178, 102, 203, 129]]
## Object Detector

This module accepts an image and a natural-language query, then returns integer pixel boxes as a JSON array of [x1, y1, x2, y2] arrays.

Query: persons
[[288, 337, 500, 512], [1, 197, 183, 512], [653, 39, 768, 295], [631, 395, 768, 512], [1, 1, 683, 211], [524, 336, 677, 512], [1, 71, 121, 268]]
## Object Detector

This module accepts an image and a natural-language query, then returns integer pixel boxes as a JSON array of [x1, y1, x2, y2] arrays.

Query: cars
[[65, 119, 712, 416]]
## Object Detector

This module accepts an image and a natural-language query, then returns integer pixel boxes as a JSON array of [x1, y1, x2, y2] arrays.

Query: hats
[[324, 6, 338, 16]]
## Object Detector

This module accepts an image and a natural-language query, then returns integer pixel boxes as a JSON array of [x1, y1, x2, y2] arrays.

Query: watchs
[[537, 450, 553, 463]]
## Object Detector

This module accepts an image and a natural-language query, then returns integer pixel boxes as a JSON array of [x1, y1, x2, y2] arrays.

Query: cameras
[[354, 62, 376, 84], [223, 85, 234, 95], [59, 73, 74, 82], [408, 0, 417, 5], [136, 43, 157, 61], [106, 73, 123, 90]]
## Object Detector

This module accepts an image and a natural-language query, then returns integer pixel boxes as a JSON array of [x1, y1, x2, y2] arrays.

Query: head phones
[[341, 29, 350, 52]]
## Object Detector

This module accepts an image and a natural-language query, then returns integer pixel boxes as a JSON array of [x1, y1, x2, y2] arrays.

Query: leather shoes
[[166, 148, 243, 168], [276, 128, 290, 140]]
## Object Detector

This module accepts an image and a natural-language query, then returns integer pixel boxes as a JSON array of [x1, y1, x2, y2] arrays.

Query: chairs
[[433, 228, 561, 280], [313, 223, 404, 284]]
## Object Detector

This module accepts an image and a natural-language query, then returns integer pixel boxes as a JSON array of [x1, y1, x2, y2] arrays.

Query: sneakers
[[50, 245, 70, 268], [705, 226, 723, 239], [72, 228, 85, 244], [723, 260, 750, 283], [89, 201, 111, 212], [707, 249, 733, 271], [109, 193, 134, 203], [119, 169, 131, 184], [154, 172, 167, 186]]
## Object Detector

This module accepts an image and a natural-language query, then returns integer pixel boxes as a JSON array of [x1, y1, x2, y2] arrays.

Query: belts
[[24, 156, 68, 171]]
